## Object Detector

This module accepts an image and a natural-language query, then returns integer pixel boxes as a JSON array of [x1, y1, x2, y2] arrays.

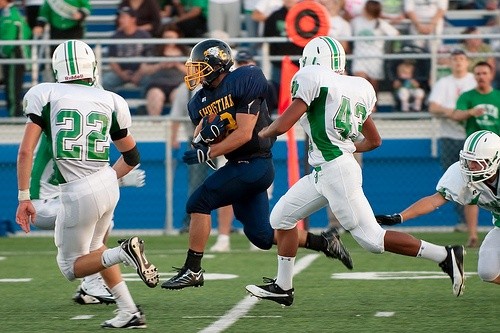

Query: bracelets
[[17, 190, 30, 200]]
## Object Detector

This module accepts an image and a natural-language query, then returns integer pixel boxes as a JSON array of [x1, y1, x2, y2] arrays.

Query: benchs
[[0, 0, 500, 119]]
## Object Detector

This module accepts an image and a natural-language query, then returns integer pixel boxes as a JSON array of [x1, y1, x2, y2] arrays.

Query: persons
[[427, 49, 478, 231], [99, 5, 154, 89], [245, 36, 466, 307], [16, 39, 160, 328], [139, 24, 192, 117], [451, 60, 500, 246], [404, 0, 449, 54], [376, 130, 500, 284], [349, 0, 401, 114], [0, 0, 500, 126], [161, 37, 353, 291], [28, 78, 147, 305]]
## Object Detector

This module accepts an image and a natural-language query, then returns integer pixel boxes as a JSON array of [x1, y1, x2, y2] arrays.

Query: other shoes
[[211, 241, 230, 253]]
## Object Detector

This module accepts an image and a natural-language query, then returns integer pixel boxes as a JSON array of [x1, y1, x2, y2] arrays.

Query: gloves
[[374, 214, 402, 226], [121, 164, 146, 188], [190, 114, 232, 150], [182, 145, 217, 172]]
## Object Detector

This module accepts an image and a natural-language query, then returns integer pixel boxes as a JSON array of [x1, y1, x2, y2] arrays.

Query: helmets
[[52, 40, 96, 83], [188, 39, 233, 88], [299, 36, 346, 72], [462, 130, 500, 184]]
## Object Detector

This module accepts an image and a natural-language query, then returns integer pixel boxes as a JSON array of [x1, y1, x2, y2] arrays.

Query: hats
[[235, 51, 251, 63], [115, 7, 136, 16]]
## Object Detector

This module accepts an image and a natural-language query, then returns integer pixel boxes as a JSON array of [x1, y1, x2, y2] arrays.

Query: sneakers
[[244, 277, 295, 307], [321, 226, 353, 270], [118, 235, 159, 288], [72, 286, 117, 305], [100, 305, 148, 329], [161, 266, 205, 290], [439, 244, 468, 297]]
